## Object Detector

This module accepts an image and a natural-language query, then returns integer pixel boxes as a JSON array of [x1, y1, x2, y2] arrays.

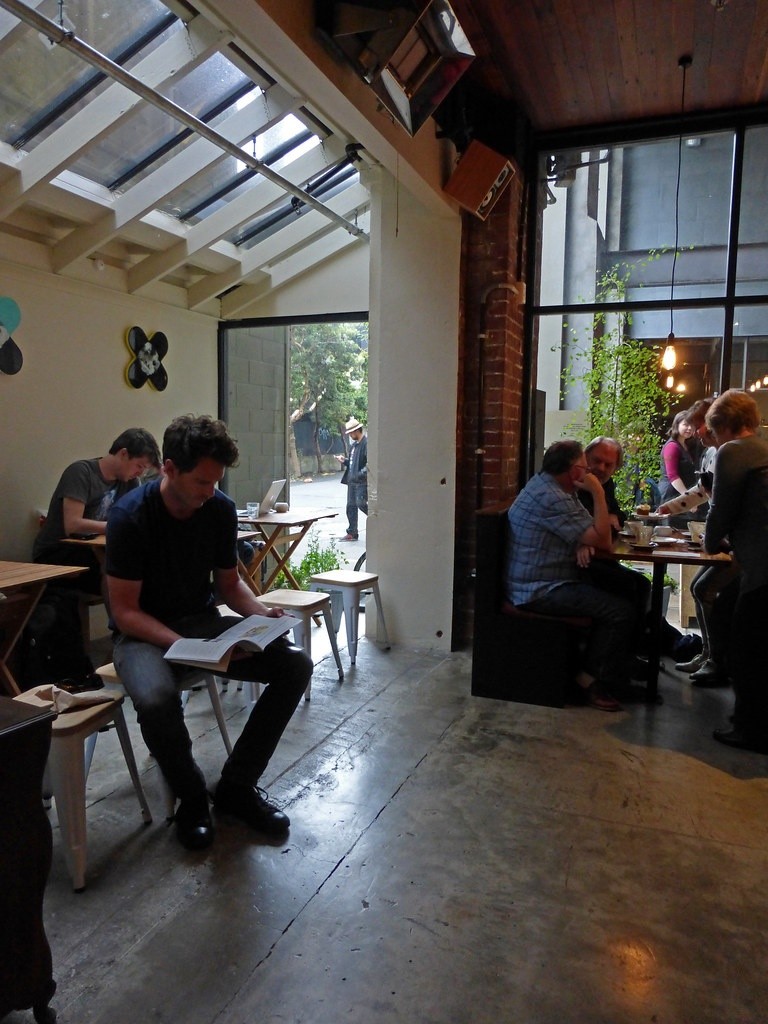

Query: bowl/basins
[[275, 502, 289, 513]]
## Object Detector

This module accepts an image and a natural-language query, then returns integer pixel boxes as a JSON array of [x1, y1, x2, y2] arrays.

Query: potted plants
[[642, 572, 680, 618]]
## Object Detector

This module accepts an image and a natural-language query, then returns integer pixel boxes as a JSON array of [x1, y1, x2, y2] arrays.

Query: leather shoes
[[712, 723, 757, 751], [688, 657, 722, 683], [675, 654, 707, 674]]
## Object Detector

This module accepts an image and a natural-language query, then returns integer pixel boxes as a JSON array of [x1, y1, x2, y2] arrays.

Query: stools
[[253, 587, 346, 702], [93, 661, 248, 825], [309, 569, 395, 665], [17, 691, 162, 892]]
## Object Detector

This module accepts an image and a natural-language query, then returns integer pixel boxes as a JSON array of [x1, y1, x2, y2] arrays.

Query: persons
[[656, 400, 725, 681], [703, 390, 768, 753], [660, 410, 699, 530], [577, 436, 651, 612], [335, 419, 367, 541], [506, 442, 652, 712], [104, 414, 312, 850], [32, 428, 161, 690]]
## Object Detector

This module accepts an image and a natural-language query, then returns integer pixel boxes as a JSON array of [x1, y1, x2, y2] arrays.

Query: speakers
[[442, 138, 516, 222]]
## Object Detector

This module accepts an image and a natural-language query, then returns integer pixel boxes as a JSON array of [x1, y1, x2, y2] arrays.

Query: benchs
[[468, 503, 604, 718]]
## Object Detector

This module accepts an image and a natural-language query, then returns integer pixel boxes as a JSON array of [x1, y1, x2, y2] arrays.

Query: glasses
[[591, 456, 618, 470], [575, 464, 592, 473]]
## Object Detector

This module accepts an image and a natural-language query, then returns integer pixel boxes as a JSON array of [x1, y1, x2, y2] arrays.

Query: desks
[[0, 559, 92, 695], [238, 507, 341, 593], [62, 530, 263, 595]]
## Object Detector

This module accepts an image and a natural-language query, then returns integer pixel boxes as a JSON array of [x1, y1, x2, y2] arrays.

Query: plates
[[686, 540, 701, 545], [650, 536, 677, 546], [631, 543, 659, 550], [681, 531, 691, 536], [618, 530, 628, 535]]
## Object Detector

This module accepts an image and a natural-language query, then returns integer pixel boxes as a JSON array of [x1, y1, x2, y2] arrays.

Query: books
[[164, 615, 301, 673]]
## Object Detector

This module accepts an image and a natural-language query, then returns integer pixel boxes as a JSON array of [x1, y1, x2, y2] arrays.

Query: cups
[[691, 522, 706, 541], [635, 526, 653, 546], [654, 526, 672, 536], [624, 520, 643, 535], [246, 502, 260, 519]]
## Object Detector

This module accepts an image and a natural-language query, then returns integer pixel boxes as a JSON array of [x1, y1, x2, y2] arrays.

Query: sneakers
[[212, 776, 290, 835], [165, 790, 218, 851], [339, 533, 358, 541]]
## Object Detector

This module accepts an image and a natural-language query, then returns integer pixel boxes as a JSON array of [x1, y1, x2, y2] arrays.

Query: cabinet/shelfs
[[0, 695, 60, 1024]]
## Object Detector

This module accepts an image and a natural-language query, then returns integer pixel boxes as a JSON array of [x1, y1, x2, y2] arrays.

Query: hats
[[344, 418, 364, 435]]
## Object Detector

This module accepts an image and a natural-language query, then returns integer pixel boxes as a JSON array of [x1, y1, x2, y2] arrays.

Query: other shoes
[[631, 654, 666, 676], [569, 677, 620, 711]]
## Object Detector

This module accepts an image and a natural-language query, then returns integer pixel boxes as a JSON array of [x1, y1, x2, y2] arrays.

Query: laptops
[[237, 479, 286, 516]]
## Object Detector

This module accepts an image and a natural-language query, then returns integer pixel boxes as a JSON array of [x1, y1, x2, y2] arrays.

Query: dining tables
[[609, 527, 742, 708]]
